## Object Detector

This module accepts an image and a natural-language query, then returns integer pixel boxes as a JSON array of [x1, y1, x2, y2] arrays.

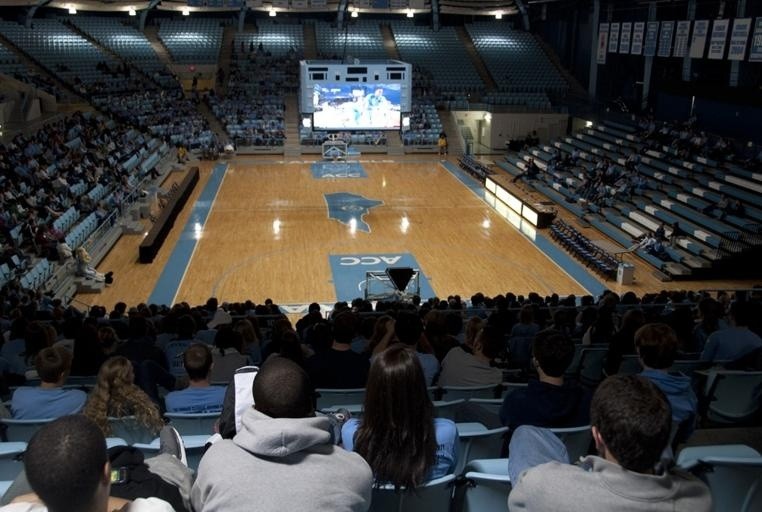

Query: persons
[[628, 220, 667, 256], [522, 129, 540, 150], [505, 370, 714, 511], [406, 65, 455, 156], [513, 157, 535, 184], [189, 353, 372, 512], [498, 331, 577, 451], [0, 414, 188, 512], [667, 221, 688, 250], [366, 87, 385, 119], [2, 286, 762, 439], [575, 147, 648, 206], [634, 114, 762, 171], [546, 146, 581, 172], [339, 345, 461, 499], [717, 200, 745, 220], [2, 37, 299, 285], [697, 193, 731, 213]]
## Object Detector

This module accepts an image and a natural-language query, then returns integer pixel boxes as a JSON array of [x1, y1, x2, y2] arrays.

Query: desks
[[479, 158, 495, 169], [591, 238, 632, 263]]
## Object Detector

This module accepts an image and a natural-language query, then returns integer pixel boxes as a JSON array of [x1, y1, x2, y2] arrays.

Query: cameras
[[109, 465, 130, 485]]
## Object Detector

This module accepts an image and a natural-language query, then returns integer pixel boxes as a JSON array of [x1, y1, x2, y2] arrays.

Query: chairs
[[501, 382, 529, 391], [299, 115, 387, 145], [455, 422, 510, 476], [563, 345, 608, 378], [105, 436, 127, 449], [204, 94, 286, 144], [131, 435, 212, 478], [0, 441, 26, 498], [548, 219, 620, 282], [326, 404, 362, 417], [617, 355, 642, 372], [0, 15, 224, 102], [316, 388, 366, 410], [369, 474, 457, 511], [702, 370, 762, 424], [430, 399, 466, 420], [675, 444, 762, 512], [460, 457, 510, 512], [468, 398, 505, 428], [426, 385, 439, 400], [456, 153, 497, 182], [108, 415, 153, 444], [0, 109, 169, 290], [0, 417, 56, 441], [228, 17, 389, 93], [163, 412, 221, 434], [674, 359, 708, 373], [389, 18, 569, 144], [89, 103, 219, 146], [442, 384, 499, 399], [569, 348, 609, 389], [548, 425, 593, 464]]
